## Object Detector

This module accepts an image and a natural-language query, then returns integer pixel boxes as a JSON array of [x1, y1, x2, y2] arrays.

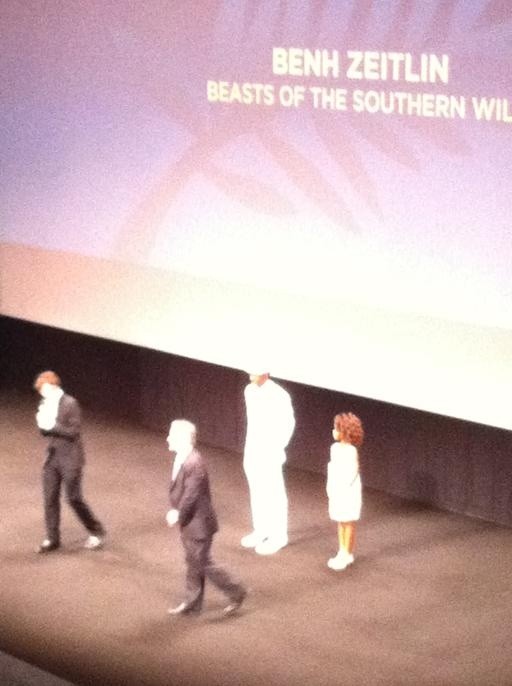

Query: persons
[[322, 411, 366, 573], [164, 419, 249, 619], [239, 357, 297, 558], [32, 370, 110, 555]]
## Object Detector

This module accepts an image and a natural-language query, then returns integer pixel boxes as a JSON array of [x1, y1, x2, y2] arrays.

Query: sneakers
[[166, 591, 244, 620], [325, 548, 356, 574], [36, 534, 105, 555], [238, 528, 288, 559]]
